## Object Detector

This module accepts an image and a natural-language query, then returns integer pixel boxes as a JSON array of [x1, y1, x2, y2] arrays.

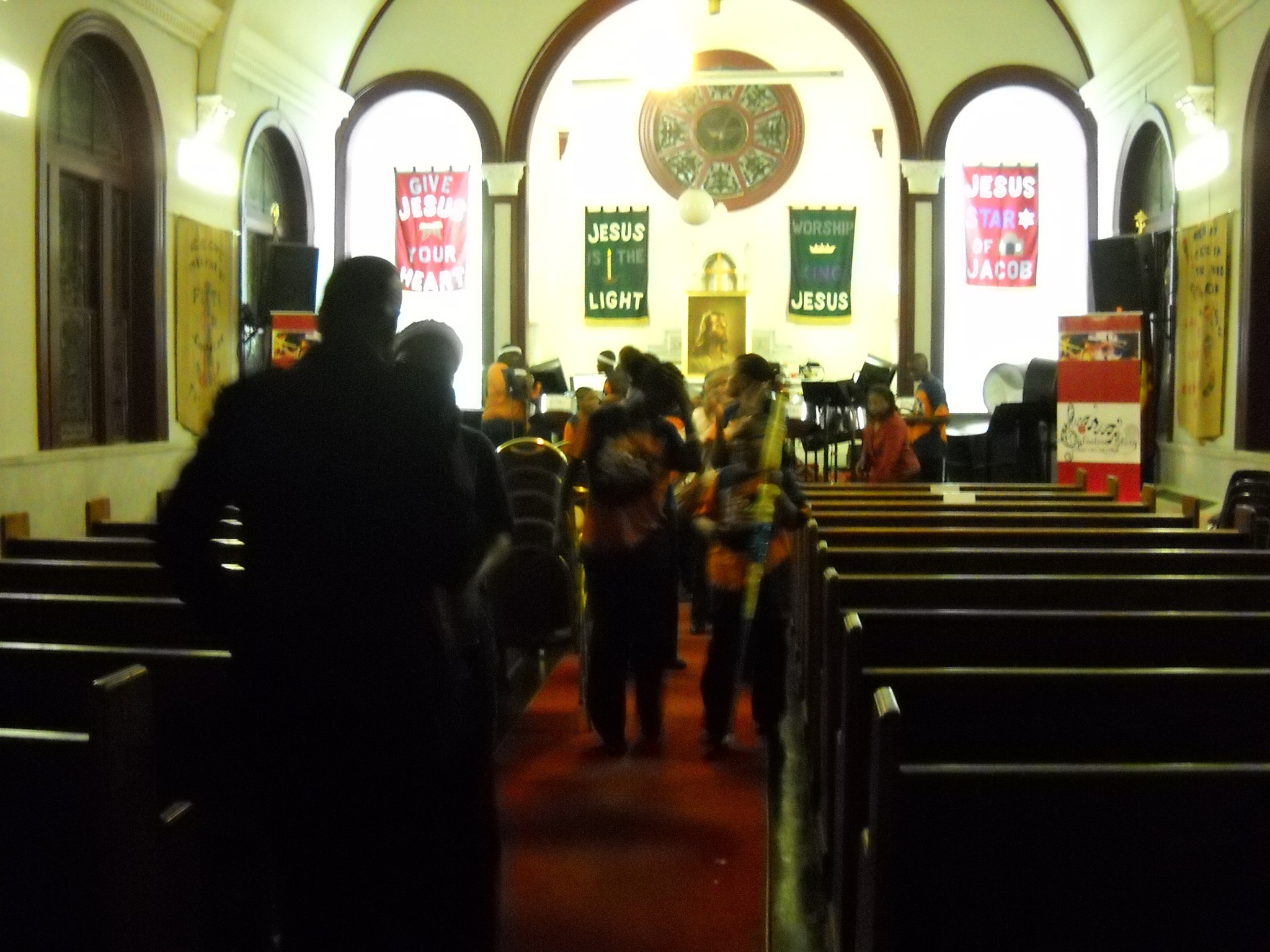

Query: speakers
[[261, 244, 318, 324], [1088, 231, 1159, 316]]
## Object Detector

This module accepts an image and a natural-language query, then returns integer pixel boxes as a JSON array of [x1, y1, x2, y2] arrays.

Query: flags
[[964, 165, 1038, 288], [395, 165, 471, 292], [584, 204, 650, 328], [785, 205, 857, 327]]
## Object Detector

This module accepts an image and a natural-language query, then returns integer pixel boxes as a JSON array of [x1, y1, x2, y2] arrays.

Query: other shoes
[[700, 716, 735, 757], [581, 738, 626, 762], [634, 726, 668, 760], [688, 611, 703, 635], [666, 659, 688, 669]]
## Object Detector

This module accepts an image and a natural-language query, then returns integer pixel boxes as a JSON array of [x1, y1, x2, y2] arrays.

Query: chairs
[[488, 437, 571, 703], [985, 357, 1058, 484]]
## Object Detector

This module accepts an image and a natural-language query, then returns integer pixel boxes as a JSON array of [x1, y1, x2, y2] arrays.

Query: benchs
[[778, 463, 1270, 950], [0, 478, 245, 946]]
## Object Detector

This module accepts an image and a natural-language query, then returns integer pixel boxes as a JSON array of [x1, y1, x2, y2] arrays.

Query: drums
[[852, 357, 896, 408], [530, 358, 568, 396]]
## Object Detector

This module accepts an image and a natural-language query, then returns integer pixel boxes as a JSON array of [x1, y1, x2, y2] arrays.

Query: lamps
[[482, 161, 526, 207], [570, 64, 845, 91], [900, 156, 942, 204]]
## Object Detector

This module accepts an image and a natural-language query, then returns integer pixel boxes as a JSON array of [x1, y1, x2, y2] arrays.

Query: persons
[[157, 255, 507, 952], [855, 384, 920, 483], [481, 344, 813, 764], [902, 353, 949, 483]]
